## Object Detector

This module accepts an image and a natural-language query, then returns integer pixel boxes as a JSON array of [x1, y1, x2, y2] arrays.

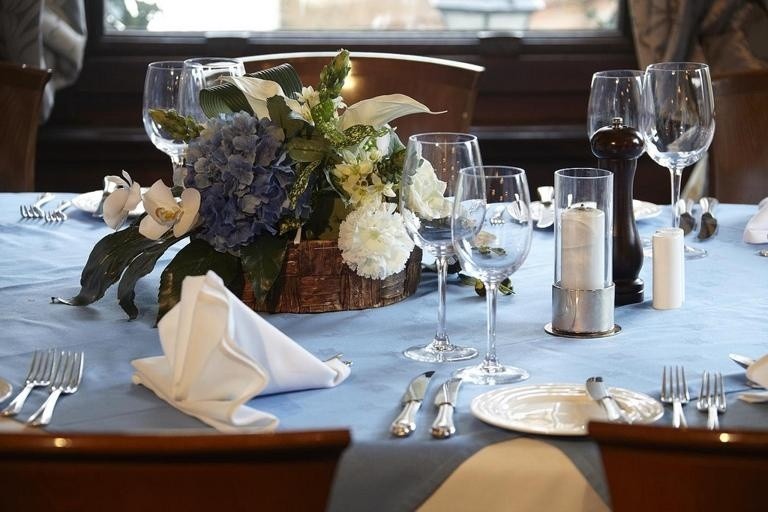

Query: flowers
[[50, 50, 514, 330]]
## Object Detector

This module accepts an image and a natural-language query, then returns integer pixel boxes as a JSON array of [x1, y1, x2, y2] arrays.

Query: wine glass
[[640, 62, 715, 227], [398, 131, 486, 366], [450, 165, 530, 384], [586, 68, 646, 161], [178, 57, 245, 126], [142, 61, 206, 171]]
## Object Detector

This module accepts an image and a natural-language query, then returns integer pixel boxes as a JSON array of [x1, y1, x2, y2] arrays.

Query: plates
[[72, 188, 148, 215], [470, 382, 664, 436]]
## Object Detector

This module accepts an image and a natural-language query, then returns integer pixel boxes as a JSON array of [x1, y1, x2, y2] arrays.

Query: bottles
[[553, 167, 615, 334], [590, 117, 646, 305], [651, 226, 683, 311]]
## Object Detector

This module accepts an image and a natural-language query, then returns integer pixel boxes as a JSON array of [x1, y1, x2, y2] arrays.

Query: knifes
[[389, 371, 436, 436], [676, 199, 695, 236], [698, 197, 718, 240], [430, 378, 463, 438], [696, 370, 727, 429], [586, 376, 627, 424], [92, 175, 119, 218], [506, 197, 662, 224], [536, 186, 556, 228], [660, 364, 690, 431]]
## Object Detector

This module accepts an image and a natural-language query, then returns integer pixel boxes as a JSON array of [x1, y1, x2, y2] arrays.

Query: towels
[[126, 270, 354, 437], [743, 195, 768, 244]]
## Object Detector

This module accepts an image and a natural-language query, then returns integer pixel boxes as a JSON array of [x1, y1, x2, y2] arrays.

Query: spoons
[[727, 353, 755, 369]]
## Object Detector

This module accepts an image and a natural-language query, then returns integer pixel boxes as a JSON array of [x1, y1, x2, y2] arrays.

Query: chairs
[[587, 420, 768, 512], [0, 60, 54, 191], [233, 51, 485, 186], [0, 428, 352, 512], [689, 67, 768, 202]]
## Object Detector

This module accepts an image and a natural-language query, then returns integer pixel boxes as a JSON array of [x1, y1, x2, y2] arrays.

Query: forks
[[44, 200, 71, 221], [25, 352, 85, 426], [1, 350, 56, 416], [20, 192, 55, 218], [490, 204, 507, 225]]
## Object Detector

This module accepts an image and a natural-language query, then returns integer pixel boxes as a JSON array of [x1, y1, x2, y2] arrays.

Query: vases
[[227, 235, 422, 315]]
